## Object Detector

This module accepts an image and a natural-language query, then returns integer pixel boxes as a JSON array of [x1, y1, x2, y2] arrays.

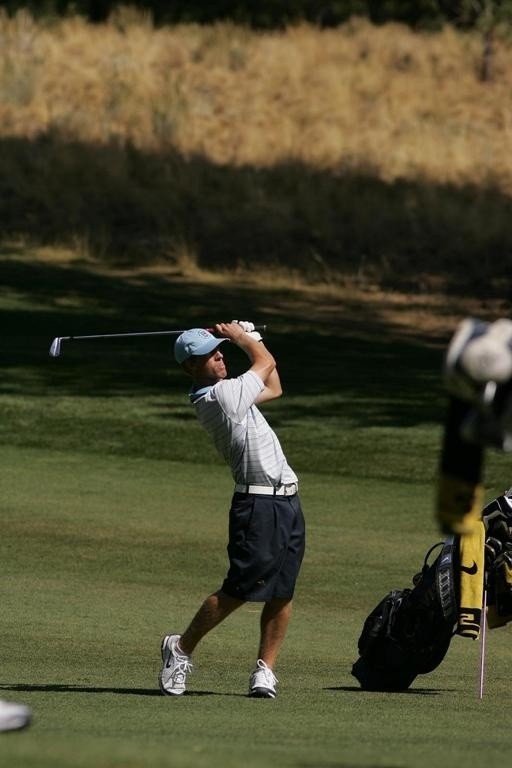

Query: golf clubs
[[488, 505, 512, 597], [50, 324, 268, 360]]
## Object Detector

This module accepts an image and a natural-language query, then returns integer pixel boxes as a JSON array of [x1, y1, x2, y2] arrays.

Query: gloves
[[231, 320, 263, 342]]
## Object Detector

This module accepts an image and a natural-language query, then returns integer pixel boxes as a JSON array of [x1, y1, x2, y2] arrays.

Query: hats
[[174, 328, 230, 363]]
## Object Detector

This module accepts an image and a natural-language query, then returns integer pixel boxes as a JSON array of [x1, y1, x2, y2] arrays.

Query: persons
[[160, 320, 304, 696]]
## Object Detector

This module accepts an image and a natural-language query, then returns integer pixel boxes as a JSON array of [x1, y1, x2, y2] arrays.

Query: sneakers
[[248, 668, 277, 699], [158, 634, 191, 697], [0, 700, 31, 732]]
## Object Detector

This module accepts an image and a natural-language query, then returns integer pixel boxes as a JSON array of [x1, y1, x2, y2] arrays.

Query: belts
[[234, 482, 298, 497]]
